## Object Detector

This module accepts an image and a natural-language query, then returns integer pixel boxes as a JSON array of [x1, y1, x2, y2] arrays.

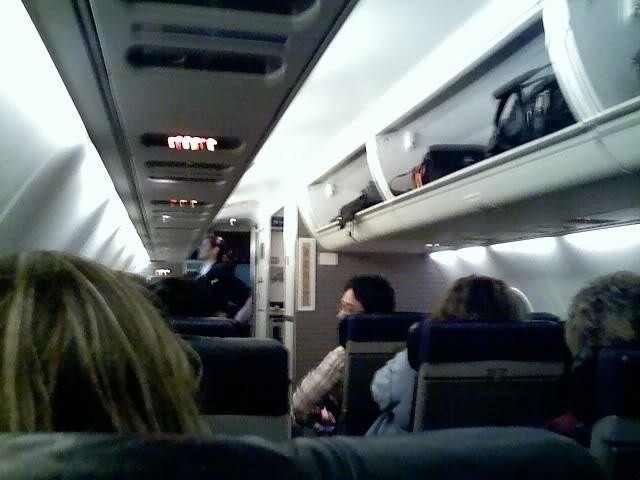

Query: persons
[[541, 272, 640, 447], [234, 295, 253, 337], [183, 235, 232, 317], [0, 249, 210, 437], [222, 249, 237, 262], [293, 275, 396, 435], [366, 276, 522, 437], [528, 312, 561, 321]]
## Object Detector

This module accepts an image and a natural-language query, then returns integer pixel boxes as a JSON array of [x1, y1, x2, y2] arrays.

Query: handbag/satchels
[[483, 61, 576, 157], [386, 140, 485, 196]]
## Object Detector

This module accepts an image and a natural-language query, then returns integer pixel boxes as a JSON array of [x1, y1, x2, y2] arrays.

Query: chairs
[[165, 318, 246, 336], [331, 311, 429, 437], [525, 313, 568, 323], [403, 322, 570, 426], [158, 334, 293, 442], [0, 434, 295, 480], [295, 426, 603, 480], [591, 416, 640, 479]]
